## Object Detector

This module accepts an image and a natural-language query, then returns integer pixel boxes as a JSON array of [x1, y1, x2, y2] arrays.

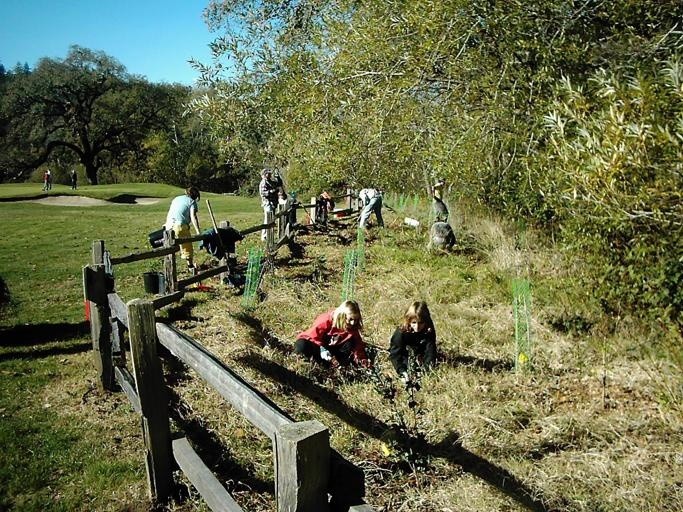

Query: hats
[[261, 168, 272, 175]]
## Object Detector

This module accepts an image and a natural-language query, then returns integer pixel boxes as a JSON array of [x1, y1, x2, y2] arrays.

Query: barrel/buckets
[[142, 272, 164, 294]]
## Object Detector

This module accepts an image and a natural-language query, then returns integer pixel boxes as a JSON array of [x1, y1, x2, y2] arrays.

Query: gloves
[[320, 346, 332, 361]]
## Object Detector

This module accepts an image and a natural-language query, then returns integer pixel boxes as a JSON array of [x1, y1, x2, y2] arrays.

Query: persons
[[41, 170, 48, 192], [294, 299, 373, 373], [353, 186, 386, 229], [71, 171, 77, 190], [163, 187, 201, 273], [387, 300, 437, 382], [44, 168, 52, 191], [257, 167, 281, 243]]
